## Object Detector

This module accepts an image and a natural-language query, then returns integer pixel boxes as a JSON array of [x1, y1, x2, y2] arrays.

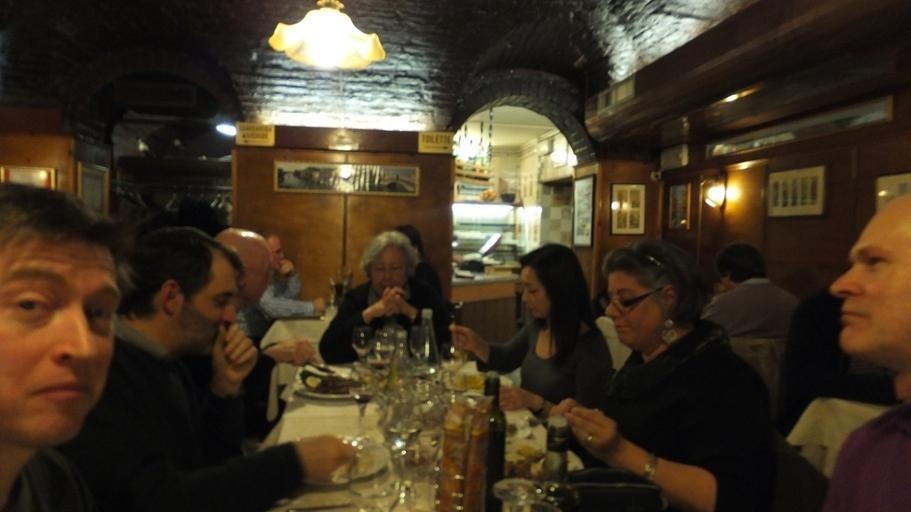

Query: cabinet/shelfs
[[454, 200, 543, 258], [452, 272, 519, 343]]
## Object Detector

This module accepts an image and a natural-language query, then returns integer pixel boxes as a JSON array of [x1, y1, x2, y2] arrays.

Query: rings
[[585, 436, 592, 445]]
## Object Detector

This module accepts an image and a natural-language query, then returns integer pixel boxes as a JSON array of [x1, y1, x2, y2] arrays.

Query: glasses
[[598, 286, 664, 313]]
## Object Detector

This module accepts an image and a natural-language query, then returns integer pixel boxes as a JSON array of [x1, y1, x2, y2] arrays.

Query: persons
[[449, 243, 613, 448], [318, 231, 452, 364], [186, 227, 319, 434], [776, 282, 911, 438], [0, 180, 121, 511], [232, 227, 326, 385], [393, 224, 443, 280], [550, 240, 770, 511], [699, 244, 801, 338], [824, 193, 911, 512], [80, 227, 355, 511]]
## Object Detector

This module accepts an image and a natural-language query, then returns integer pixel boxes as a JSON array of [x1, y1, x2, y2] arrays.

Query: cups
[[376, 331, 394, 364], [347, 367, 376, 419]]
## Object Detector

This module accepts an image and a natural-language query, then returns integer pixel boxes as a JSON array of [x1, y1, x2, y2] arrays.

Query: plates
[[291, 381, 353, 399]]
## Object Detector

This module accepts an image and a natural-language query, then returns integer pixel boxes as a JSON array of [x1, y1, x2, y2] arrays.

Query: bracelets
[[639, 454, 658, 482], [535, 395, 547, 417]]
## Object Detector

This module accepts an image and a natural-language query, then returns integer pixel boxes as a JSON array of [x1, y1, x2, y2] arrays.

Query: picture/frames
[[669, 182, 691, 231], [272, 160, 421, 197], [572, 172, 597, 249], [764, 165, 828, 216], [610, 180, 647, 236], [874, 173, 910, 208]]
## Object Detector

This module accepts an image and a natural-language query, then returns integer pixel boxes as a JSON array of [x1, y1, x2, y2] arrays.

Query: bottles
[[544, 417, 569, 483], [415, 308, 440, 377], [482, 371, 507, 511]]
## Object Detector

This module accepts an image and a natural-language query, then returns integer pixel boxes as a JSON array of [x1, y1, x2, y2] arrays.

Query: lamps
[[267, 0, 386, 71], [215, 113, 237, 139]]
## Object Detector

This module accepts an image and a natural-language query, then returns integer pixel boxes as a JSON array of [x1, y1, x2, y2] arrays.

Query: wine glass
[[409, 322, 436, 379], [351, 326, 373, 364], [442, 342, 465, 402], [349, 442, 401, 512]]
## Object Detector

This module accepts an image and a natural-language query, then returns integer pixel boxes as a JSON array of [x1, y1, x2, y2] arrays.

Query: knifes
[[307, 360, 335, 372]]
